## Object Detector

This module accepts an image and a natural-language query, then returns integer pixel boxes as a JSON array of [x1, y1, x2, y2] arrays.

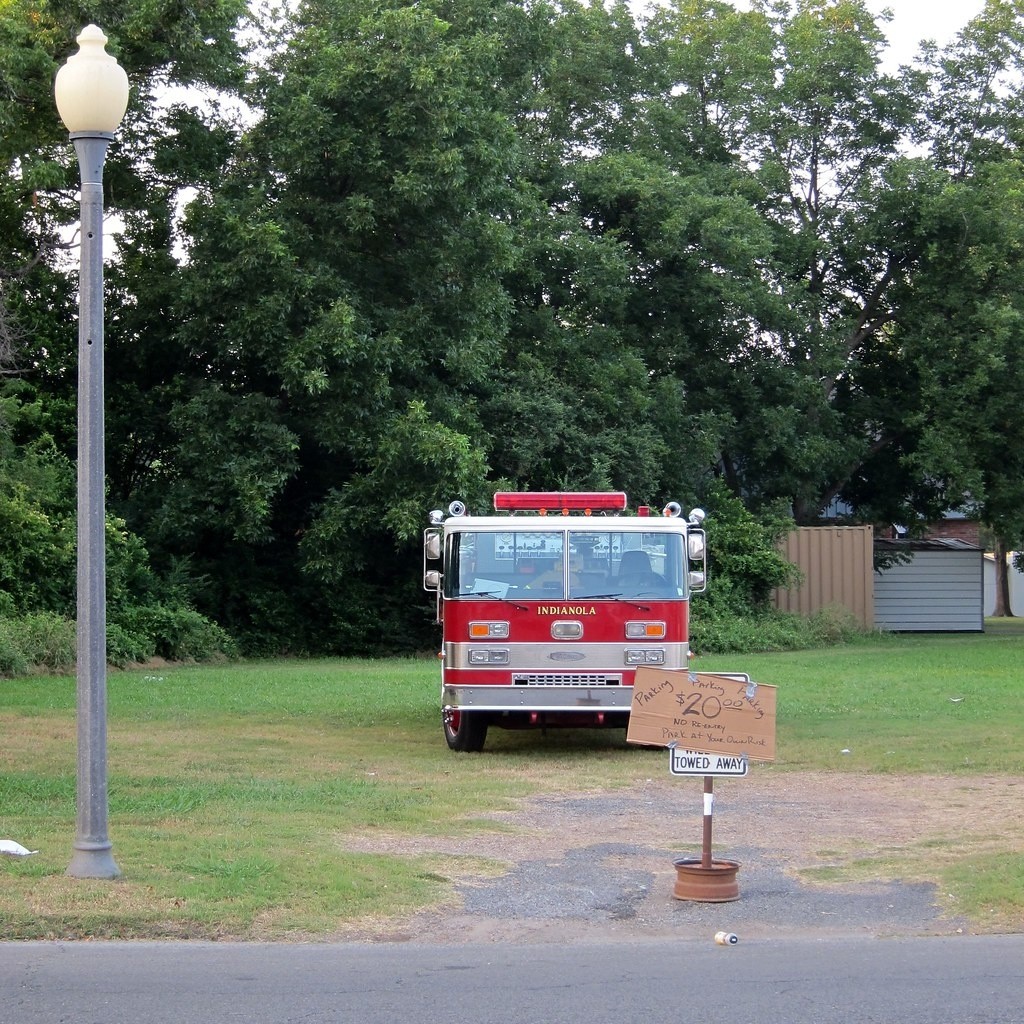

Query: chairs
[[554, 553, 582, 573], [618, 551, 653, 588]]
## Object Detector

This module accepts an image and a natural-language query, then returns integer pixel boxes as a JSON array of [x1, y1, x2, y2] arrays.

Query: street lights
[[52, 26, 135, 880]]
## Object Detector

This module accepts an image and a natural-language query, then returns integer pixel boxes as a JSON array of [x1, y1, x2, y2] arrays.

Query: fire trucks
[[418, 488, 715, 752]]
